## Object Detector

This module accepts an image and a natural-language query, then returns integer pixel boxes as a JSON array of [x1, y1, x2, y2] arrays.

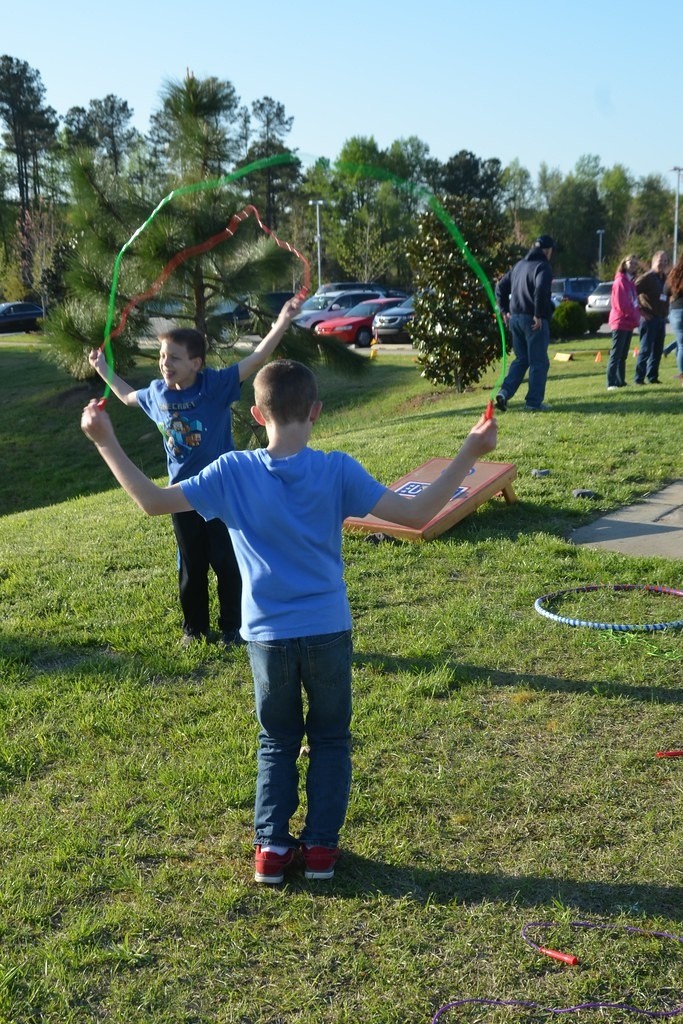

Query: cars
[[552, 294, 566, 309], [373, 286, 439, 343], [314, 296, 407, 349], [0, 302, 44, 334]]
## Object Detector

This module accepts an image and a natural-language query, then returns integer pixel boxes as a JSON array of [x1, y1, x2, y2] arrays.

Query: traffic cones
[[633, 346, 639, 357], [594, 351, 603, 363]]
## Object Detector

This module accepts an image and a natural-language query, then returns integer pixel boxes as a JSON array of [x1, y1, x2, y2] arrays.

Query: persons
[[606, 250, 683, 390], [494, 233, 555, 412], [81, 399, 499, 883], [89, 298, 304, 646]]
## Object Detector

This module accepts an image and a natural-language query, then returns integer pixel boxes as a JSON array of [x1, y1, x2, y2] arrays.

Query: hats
[[535, 235, 554, 247]]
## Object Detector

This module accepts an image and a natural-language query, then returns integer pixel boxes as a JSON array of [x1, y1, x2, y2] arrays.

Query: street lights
[[669, 166, 683, 267], [596, 229, 605, 265], [308, 199, 337, 286]]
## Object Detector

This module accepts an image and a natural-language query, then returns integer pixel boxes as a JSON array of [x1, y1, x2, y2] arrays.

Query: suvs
[[207, 291, 296, 344], [293, 291, 391, 331], [316, 282, 385, 290], [547, 277, 604, 301], [585, 279, 615, 312]]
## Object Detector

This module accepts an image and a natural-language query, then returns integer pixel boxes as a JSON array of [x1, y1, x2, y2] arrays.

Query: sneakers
[[524, 404, 553, 412], [496, 393, 507, 411], [300, 844, 340, 879], [254, 845, 295, 883], [223, 628, 240, 653], [181, 632, 202, 648]]
